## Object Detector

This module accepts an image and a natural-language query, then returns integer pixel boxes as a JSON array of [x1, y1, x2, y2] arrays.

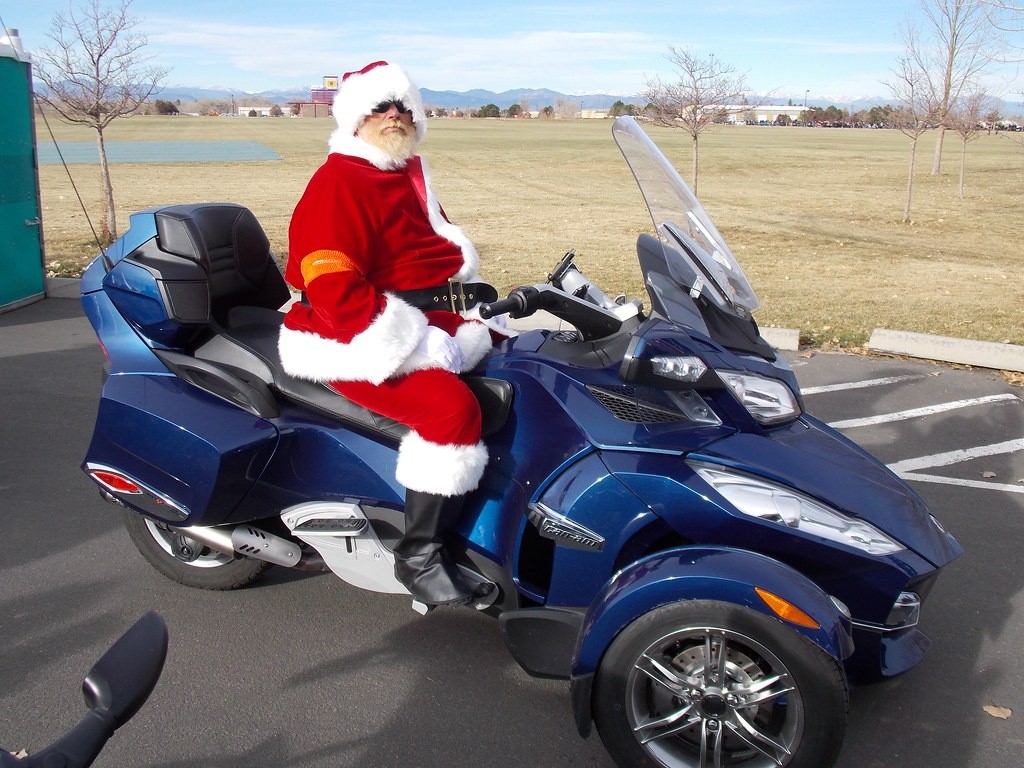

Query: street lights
[[804, 90, 810, 121], [581, 101, 583, 118], [231, 94, 235, 116]]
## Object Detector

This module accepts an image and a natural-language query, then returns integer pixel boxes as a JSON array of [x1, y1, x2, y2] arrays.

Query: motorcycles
[[0, 610, 169, 768], [79, 116, 963, 768]]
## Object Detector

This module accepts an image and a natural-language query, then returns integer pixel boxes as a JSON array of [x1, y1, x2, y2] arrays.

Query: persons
[[278, 60, 507, 606]]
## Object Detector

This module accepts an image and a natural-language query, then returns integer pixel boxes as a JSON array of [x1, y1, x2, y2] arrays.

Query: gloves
[[415, 326, 466, 374]]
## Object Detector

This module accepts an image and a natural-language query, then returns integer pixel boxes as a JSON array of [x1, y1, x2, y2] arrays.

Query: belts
[[301, 281, 497, 314]]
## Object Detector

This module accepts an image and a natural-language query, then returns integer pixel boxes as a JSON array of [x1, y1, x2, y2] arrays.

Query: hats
[[330, 61, 428, 144]]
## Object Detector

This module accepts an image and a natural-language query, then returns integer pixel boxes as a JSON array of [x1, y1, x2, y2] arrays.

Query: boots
[[393, 429, 489, 605]]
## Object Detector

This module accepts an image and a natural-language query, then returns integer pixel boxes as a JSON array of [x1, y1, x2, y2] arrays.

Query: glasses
[[373, 101, 409, 114]]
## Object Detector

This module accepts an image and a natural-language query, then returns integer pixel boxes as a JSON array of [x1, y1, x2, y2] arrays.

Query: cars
[[225, 113, 233, 117], [230, 114, 239, 117], [289, 114, 300, 118], [219, 113, 226, 118], [707, 119, 1024, 132], [260, 115, 267, 117]]
